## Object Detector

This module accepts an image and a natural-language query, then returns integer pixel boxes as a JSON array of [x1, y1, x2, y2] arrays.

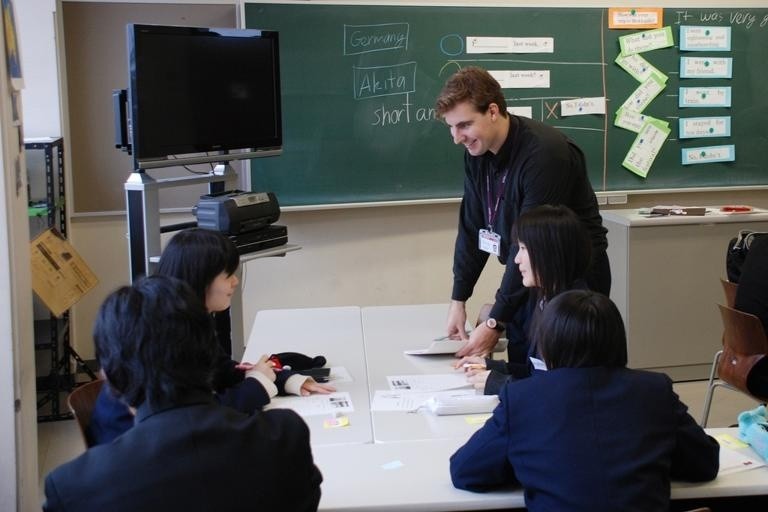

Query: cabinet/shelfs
[[24, 136, 91, 422]]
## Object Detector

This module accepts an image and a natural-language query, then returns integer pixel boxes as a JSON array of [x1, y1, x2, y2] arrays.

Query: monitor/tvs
[[125, 23, 283, 160]]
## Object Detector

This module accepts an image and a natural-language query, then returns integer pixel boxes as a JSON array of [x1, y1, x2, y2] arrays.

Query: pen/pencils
[[235, 364, 284, 372], [464, 362, 487, 370], [434, 331, 473, 341]]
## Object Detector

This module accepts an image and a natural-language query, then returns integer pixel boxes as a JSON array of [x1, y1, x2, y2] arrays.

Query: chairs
[[68, 381, 107, 452], [702, 229, 768, 428]]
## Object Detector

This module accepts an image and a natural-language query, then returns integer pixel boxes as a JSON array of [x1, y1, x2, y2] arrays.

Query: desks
[[229, 304, 532, 512], [597, 206, 768, 382], [666, 419, 768, 511]]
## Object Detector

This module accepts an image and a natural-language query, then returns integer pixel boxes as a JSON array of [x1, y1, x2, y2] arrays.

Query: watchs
[[487, 318, 506, 332]]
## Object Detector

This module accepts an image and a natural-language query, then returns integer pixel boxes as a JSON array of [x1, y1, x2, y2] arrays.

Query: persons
[[38, 273, 328, 510], [429, 65, 615, 376], [86, 226, 339, 414], [442, 286, 726, 510], [451, 204, 595, 396]]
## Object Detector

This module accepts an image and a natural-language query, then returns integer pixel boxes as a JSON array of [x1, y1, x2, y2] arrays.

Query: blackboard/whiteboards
[[236, 0, 767, 213]]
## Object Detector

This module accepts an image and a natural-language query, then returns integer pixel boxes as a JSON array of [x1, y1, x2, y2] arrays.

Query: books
[[650, 204, 706, 217], [404, 337, 510, 356], [427, 384, 501, 417]]
